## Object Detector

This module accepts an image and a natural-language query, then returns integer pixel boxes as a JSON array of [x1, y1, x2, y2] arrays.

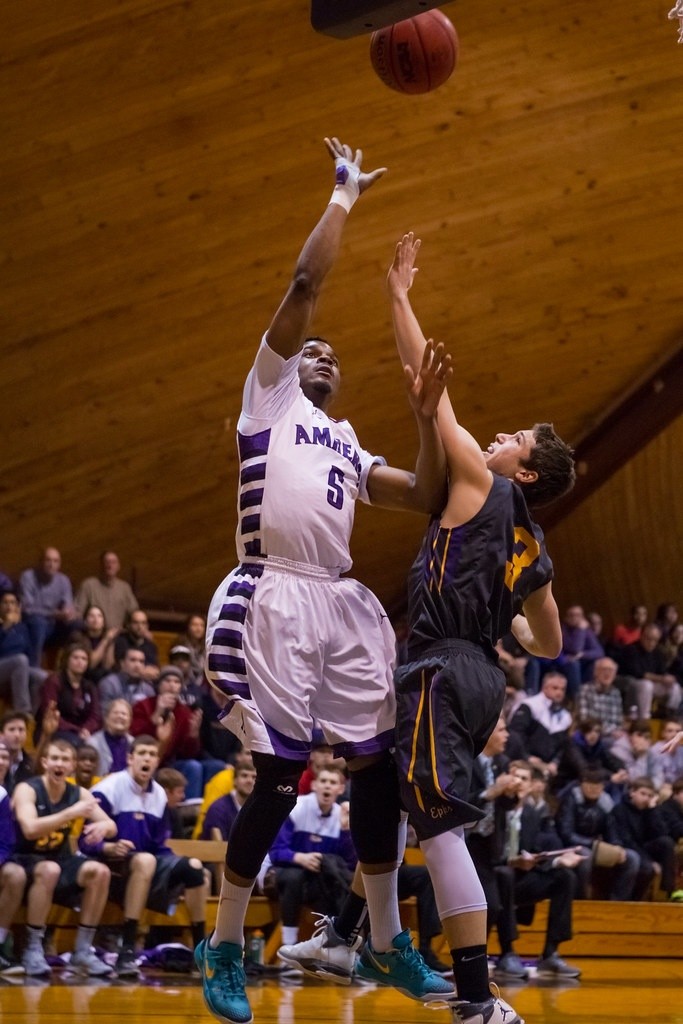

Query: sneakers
[[277, 912, 363, 985], [419, 947, 497, 978], [22, 950, 52, 975], [535, 952, 582, 977], [195, 925, 254, 1024], [69, 949, 113, 976], [447, 981, 525, 1024], [115, 956, 141, 976], [354, 934, 457, 1009], [280, 961, 305, 978], [0, 951, 25, 974], [494, 952, 529, 978]]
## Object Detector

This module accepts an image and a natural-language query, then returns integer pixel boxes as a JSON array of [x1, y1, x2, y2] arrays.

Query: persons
[[0, 547, 683, 981], [195, 134, 456, 1024], [284, 232, 575, 1024]]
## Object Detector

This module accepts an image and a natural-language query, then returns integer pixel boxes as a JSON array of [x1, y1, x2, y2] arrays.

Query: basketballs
[[369, 8, 459, 96]]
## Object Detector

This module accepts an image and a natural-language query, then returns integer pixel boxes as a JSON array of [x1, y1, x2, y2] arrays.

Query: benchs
[[1, 634, 682, 956]]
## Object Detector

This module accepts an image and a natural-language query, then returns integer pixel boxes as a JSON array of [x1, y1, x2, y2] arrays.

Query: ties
[[481, 760, 495, 837]]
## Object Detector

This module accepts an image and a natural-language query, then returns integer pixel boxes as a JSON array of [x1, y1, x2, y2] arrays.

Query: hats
[[171, 645, 189, 658], [582, 763, 606, 784], [154, 667, 184, 685]]
